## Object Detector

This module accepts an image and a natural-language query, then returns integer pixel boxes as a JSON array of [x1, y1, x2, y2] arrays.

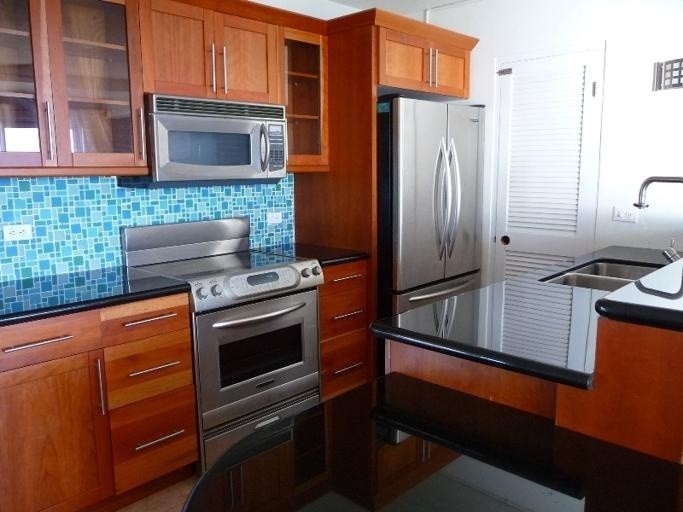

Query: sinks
[[542, 273, 635, 292], [571, 261, 658, 280]]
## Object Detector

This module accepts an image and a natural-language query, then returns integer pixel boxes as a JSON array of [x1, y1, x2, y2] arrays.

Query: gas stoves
[[123, 217, 324, 312]]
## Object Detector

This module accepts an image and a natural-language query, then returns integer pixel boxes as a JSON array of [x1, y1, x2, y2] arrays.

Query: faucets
[[636, 267, 683, 300], [634, 176, 682, 260]]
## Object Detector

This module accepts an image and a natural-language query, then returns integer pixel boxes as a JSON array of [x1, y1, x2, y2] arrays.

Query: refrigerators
[[378, 93, 486, 445]]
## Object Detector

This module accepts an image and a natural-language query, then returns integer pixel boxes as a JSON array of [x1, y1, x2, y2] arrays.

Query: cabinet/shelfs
[[139, 0, 279, 114], [100, 285, 199, 510], [278, 26, 328, 174], [0, 0, 150, 176], [0, 292, 112, 512], [371, 246, 682, 464], [378, 26, 471, 102], [318, 255, 374, 402]]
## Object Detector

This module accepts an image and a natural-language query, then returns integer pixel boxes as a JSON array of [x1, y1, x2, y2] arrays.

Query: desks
[[183, 371, 683, 512]]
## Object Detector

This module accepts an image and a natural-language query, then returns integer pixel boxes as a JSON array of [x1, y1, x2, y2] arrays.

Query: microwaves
[[149, 95, 289, 182]]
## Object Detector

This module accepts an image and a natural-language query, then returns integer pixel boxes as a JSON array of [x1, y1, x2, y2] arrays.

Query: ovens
[[195, 290, 322, 475]]
